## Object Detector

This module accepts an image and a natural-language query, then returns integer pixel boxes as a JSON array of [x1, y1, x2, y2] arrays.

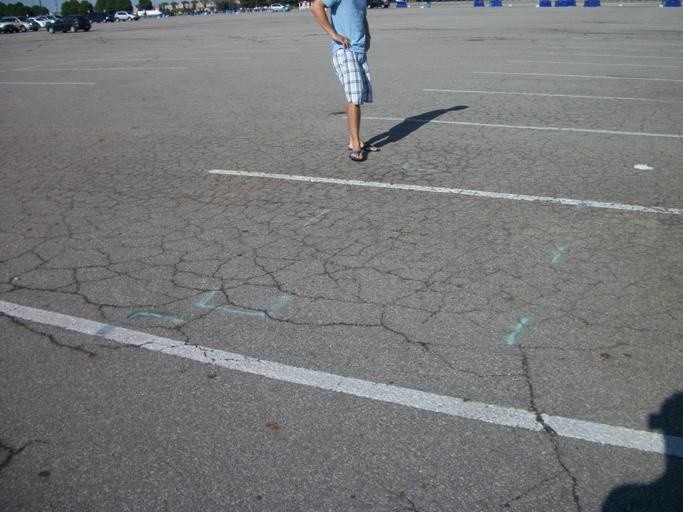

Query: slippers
[[348, 143, 380, 161]]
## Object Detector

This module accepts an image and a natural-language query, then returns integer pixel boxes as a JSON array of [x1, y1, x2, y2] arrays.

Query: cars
[[269, 2, 285, 12], [365, 0, 389, 8], [0, 9, 140, 34]]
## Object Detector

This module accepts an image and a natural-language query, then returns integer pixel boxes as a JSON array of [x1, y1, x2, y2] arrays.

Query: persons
[[308, 0, 380, 162]]
